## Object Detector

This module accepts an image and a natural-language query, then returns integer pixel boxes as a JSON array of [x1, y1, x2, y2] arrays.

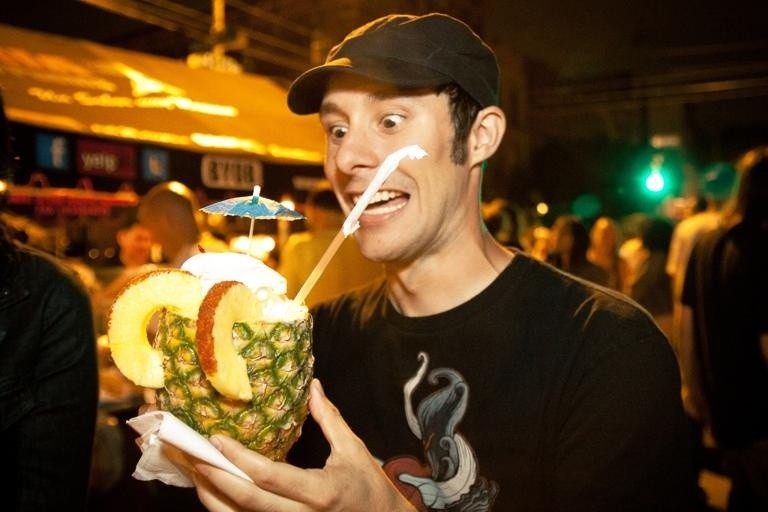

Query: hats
[[286, 12, 500, 114]]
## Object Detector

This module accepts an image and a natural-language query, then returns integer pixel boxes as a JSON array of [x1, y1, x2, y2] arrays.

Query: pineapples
[[106, 248, 316, 463]]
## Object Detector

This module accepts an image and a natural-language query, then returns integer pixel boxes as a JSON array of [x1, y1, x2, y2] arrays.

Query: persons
[[158, 14, 703, 512], [0, 170, 389, 510], [481, 149, 767, 512]]
